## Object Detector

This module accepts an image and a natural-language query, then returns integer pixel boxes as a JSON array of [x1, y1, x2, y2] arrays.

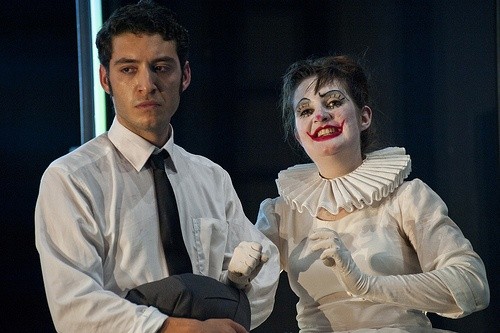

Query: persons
[[34, 0, 279, 333], [218, 54, 491, 333]]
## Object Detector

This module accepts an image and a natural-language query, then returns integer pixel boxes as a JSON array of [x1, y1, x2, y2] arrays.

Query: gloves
[[308, 227, 369, 297], [227, 240, 269, 284]]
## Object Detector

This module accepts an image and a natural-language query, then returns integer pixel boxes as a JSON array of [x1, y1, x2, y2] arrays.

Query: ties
[[144, 149, 193, 277]]
